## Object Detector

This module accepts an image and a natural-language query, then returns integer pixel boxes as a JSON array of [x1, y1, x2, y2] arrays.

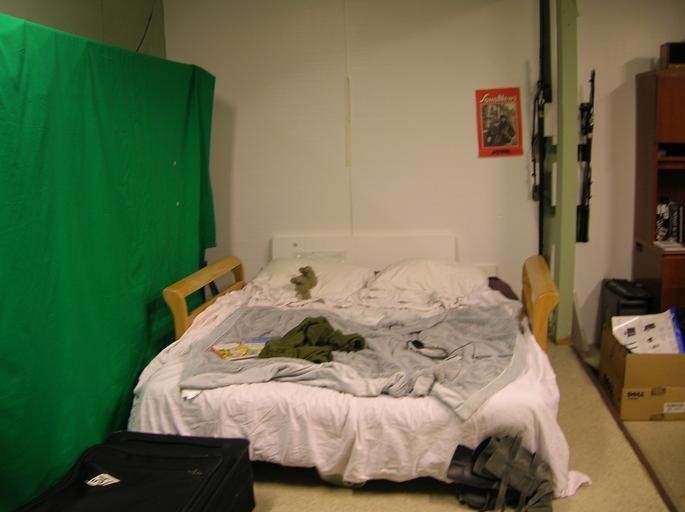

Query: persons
[[484, 119, 500, 146], [497, 115, 515, 145]]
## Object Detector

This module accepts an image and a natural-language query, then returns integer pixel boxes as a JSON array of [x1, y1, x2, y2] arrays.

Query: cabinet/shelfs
[[634, 71, 685, 248], [632, 244, 685, 333]]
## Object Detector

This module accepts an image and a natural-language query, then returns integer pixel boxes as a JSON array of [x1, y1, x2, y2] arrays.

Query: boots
[[447, 435, 554, 512]]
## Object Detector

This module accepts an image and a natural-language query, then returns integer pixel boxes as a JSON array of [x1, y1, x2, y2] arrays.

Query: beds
[[128, 233, 559, 498]]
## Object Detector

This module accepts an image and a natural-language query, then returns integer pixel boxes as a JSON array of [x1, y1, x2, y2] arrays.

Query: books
[[653, 201, 685, 253], [213, 335, 282, 361]]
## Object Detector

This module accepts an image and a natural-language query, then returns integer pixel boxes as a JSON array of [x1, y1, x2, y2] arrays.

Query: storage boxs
[[597, 321, 685, 420]]
